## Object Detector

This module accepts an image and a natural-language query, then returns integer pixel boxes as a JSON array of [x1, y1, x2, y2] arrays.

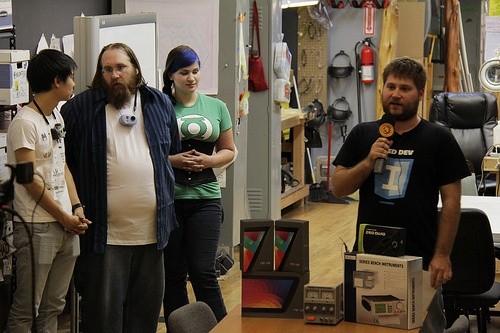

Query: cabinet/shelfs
[[281, 109, 315, 210]]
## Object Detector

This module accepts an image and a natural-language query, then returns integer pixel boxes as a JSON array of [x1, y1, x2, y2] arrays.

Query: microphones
[[373, 113, 396, 174]]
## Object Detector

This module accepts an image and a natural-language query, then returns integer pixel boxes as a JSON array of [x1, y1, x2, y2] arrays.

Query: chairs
[[435, 207, 500, 333], [168, 301, 218, 333], [429, 92, 498, 196]]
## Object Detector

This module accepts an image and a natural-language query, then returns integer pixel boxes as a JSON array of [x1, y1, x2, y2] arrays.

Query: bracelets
[[72, 203, 86, 213]]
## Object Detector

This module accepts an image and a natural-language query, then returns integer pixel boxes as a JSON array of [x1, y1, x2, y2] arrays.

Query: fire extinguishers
[[355, 37, 379, 83]]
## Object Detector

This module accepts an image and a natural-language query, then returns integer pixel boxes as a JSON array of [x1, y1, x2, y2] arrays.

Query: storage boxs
[[0, 49, 30, 105], [0, 0, 14, 29], [0, 132, 14, 275], [343, 224, 425, 329], [240, 219, 310, 319]]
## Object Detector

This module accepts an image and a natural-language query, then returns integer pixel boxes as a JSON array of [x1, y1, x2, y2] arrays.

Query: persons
[[6, 49, 92, 333], [331, 58, 471, 333], [60, 43, 181, 333], [162, 45, 234, 333]]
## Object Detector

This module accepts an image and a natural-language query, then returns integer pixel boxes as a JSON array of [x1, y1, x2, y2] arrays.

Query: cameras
[[51, 123, 67, 140]]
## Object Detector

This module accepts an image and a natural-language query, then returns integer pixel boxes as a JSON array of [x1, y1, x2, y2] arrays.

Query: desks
[[437, 194, 500, 248], [207, 304, 429, 333]]
